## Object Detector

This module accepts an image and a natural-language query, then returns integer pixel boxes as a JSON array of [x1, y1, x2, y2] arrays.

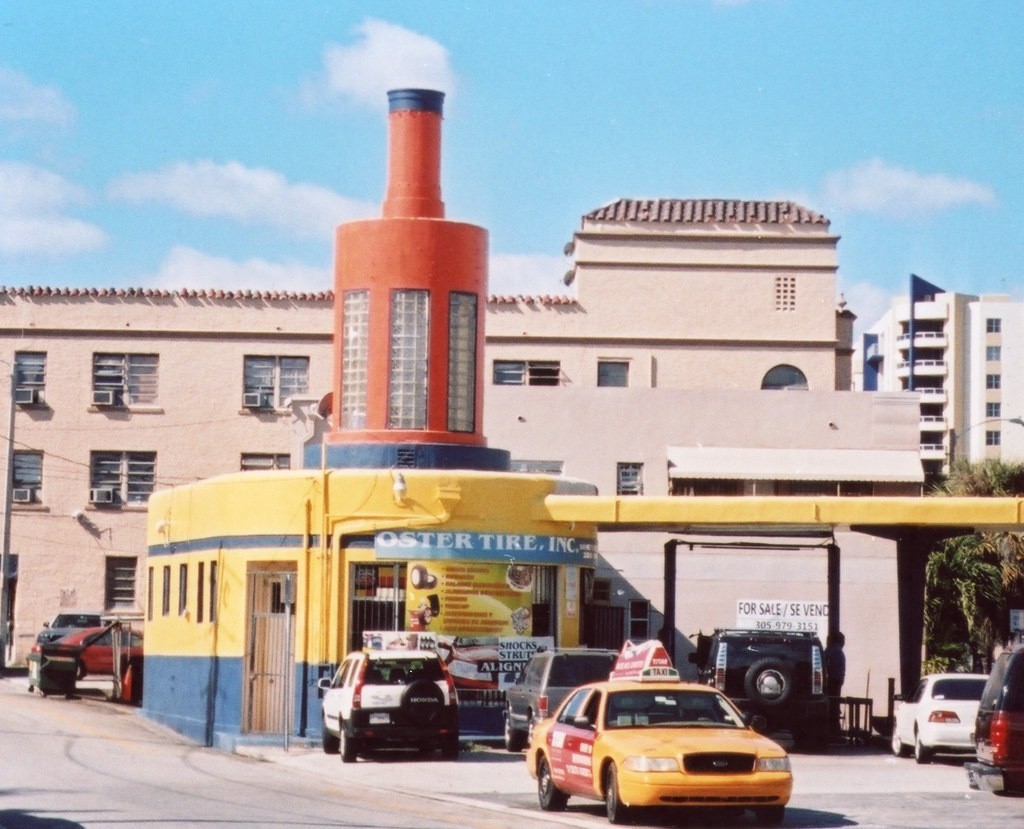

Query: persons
[[824, 632, 846, 744]]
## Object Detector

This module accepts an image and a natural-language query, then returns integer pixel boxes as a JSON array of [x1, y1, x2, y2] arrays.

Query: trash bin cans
[[25, 643, 86, 697]]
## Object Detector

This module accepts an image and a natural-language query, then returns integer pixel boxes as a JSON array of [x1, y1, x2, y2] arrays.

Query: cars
[[28, 625, 144, 682], [503, 648, 619, 754], [36, 612, 101, 644], [523, 638, 792, 829], [890, 672, 990, 760]]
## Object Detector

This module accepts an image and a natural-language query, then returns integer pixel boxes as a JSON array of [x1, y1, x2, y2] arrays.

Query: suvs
[[317, 648, 459, 763], [963, 643, 1024, 800], [691, 628, 833, 754]]
